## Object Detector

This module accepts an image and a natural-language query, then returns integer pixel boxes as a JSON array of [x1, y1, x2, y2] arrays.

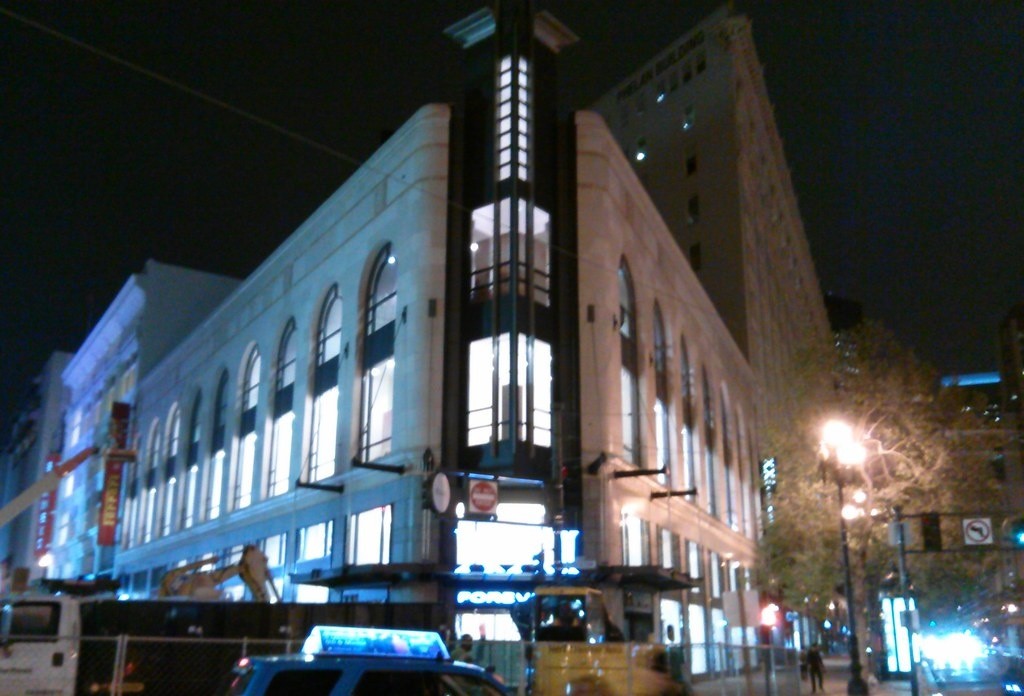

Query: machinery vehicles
[[0, 430, 137, 533], [157, 545, 281, 604]]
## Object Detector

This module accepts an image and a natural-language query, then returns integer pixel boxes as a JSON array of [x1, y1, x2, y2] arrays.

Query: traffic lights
[[1010, 518, 1024, 545], [922, 516, 941, 550]]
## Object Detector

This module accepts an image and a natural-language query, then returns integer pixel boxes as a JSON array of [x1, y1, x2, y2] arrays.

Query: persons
[[666, 625, 675, 644], [799, 646, 808, 682], [806, 643, 825, 695], [451, 634, 473, 663]]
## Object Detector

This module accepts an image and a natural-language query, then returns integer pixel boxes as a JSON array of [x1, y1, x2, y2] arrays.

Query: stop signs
[[470, 477, 499, 515]]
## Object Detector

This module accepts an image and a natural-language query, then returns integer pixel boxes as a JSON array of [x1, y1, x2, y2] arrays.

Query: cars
[[0, 592, 82, 695], [219, 627, 507, 696]]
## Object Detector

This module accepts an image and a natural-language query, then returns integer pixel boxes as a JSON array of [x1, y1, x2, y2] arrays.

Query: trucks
[[531, 587, 685, 695]]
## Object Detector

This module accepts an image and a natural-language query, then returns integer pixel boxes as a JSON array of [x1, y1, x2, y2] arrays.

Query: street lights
[[823, 418, 863, 665]]
